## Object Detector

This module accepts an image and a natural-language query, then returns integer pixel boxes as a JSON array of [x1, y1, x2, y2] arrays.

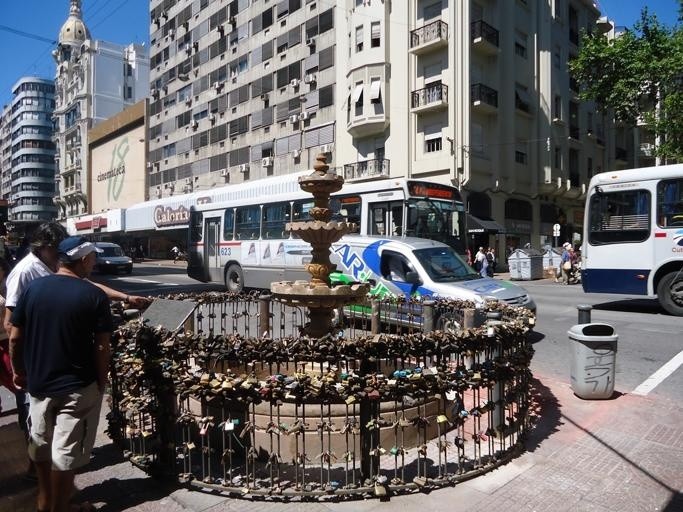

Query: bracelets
[[127, 295, 130, 302]]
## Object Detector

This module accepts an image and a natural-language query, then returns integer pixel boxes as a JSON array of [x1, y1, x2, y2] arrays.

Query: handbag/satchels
[[564, 262, 571, 270]]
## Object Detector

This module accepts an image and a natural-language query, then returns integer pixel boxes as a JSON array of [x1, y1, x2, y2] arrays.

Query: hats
[[56, 236, 104, 263]]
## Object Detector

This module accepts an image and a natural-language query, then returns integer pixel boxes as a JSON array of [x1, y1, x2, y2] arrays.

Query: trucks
[[80, 233, 112, 243]]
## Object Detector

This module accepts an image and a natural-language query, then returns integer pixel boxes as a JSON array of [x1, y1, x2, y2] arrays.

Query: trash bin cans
[[567, 323, 619, 400]]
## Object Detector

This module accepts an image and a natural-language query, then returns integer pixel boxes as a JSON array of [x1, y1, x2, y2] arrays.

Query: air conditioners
[[145, 9, 331, 198]]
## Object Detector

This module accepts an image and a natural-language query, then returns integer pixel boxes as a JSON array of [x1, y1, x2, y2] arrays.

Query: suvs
[[90, 242, 132, 276]]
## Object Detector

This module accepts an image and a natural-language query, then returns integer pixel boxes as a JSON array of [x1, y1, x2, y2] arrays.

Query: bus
[[185, 178, 472, 295], [580, 164, 683, 317]]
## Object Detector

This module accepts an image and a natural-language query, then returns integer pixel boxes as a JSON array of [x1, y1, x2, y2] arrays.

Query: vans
[[327, 234, 536, 338]]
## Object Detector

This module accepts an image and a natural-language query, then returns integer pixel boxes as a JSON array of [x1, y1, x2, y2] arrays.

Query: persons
[[465, 241, 580, 285], [170, 246, 179, 260], [16, 234, 32, 259], [3, 221, 153, 446], [7, 234, 114, 512]]
